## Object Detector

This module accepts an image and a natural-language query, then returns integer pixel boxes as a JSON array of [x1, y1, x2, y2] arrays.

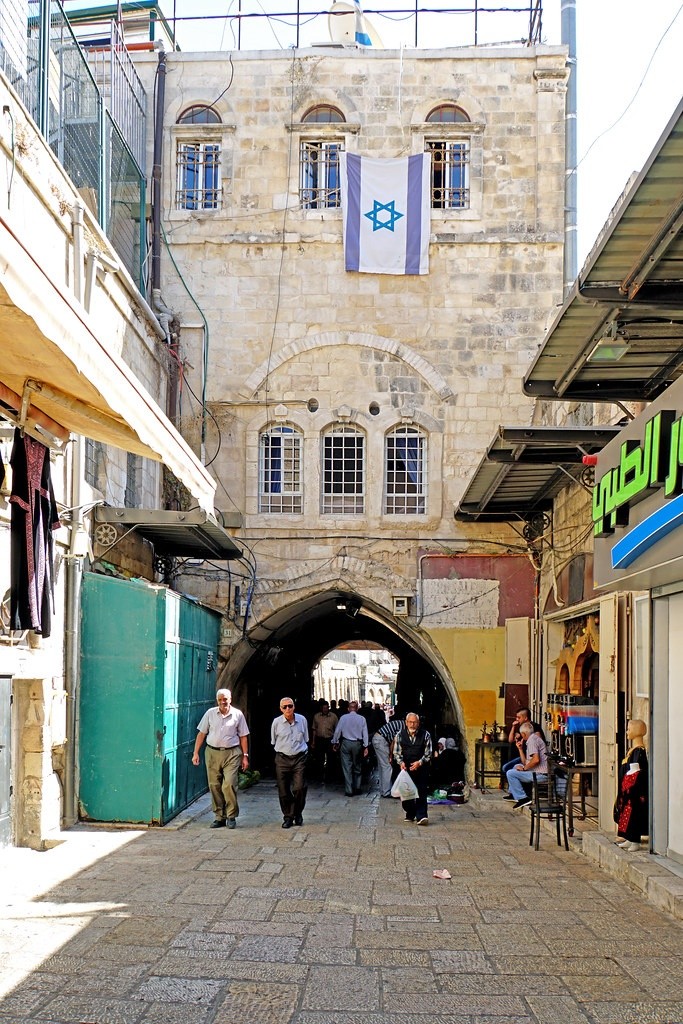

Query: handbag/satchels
[[391, 769, 419, 801]]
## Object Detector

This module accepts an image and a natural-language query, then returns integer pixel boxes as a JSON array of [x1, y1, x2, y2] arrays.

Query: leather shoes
[[295, 817, 302, 825], [282, 818, 293, 828]]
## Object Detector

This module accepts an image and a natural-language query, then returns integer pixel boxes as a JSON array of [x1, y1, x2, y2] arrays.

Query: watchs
[[243, 753, 248, 757]]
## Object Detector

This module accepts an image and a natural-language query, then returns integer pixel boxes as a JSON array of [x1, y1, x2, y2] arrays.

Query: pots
[[447, 794, 465, 804]]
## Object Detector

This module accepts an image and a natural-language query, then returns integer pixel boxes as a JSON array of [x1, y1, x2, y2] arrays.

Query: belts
[[207, 744, 239, 751]]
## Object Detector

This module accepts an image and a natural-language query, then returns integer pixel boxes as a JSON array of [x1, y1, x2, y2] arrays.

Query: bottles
[[484, 735, 489, 743], [498, 726, 507, 741]]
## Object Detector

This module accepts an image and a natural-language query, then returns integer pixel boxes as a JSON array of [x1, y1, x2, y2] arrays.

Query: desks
[[546, 757, 598, 836], [474, 738, 512, 794]]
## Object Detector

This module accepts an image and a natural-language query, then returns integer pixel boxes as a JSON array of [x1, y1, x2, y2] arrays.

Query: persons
[[192, 689, 249, 828], [617, 719, 648, 851], [271, 697, 309, 827], [394, 712, 432, 825], [501, 708, 546, 802], [304, 698, 467, 797], [506, 723, 548, 809]]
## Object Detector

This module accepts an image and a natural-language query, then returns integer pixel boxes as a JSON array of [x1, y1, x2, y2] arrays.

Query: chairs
[[529, 772, 571, 851]]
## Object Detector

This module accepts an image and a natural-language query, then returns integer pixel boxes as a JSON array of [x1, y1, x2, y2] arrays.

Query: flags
[[338, 152, 431, 275]]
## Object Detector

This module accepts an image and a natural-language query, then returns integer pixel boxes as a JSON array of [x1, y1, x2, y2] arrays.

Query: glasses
[[280, 704, 293, 709]]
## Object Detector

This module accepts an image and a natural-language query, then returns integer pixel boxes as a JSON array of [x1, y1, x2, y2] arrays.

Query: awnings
[[0, 205, 216, 519], [521, 97, 683, 403], [91, 504, 242, 563], [453, 425, 623, 523]]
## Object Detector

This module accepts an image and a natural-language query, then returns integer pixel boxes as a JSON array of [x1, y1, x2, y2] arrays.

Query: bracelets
[[523, 766, 524, 771]]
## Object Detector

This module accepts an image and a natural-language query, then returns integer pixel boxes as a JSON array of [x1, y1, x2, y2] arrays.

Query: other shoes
[[210, 819, 226, 828], [345, 793, 354, 797], [356, 790, 362, 795], [382, 794, 396, 799], [404, 815, 414, 822], [227, 819, 236, 828], [417, 816, 429, 825], [502, 794, 519, 802], [513, 797, 532, 810]]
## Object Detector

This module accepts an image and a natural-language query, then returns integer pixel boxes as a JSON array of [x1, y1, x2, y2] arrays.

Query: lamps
[[337, 601, 347, 610]]
